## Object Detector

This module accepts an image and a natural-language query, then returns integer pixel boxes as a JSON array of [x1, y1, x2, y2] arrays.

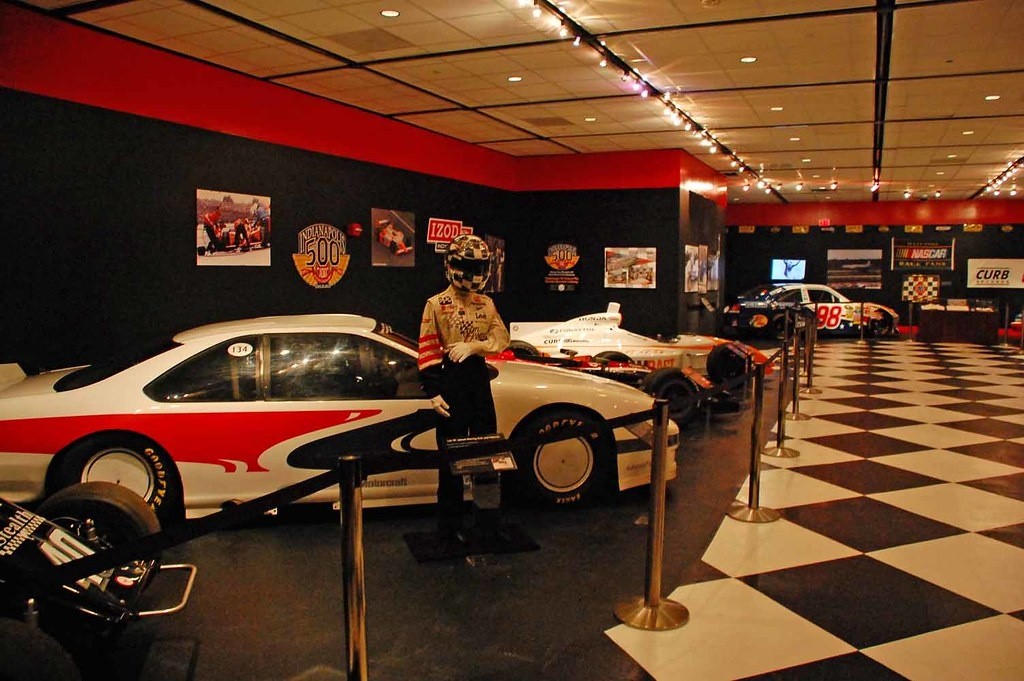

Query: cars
[[723, 284, 899, 337], [0, 496, 197, 681]]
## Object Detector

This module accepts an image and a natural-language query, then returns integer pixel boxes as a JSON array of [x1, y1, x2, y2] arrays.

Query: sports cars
[[511, 353, 748, 425], [0, 311, 679, 536], [507, 309, 774, 390]]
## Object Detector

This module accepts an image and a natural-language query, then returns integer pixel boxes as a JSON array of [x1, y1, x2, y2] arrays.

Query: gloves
[[430, 395, 450, 418], [445, 341, 470, 363]]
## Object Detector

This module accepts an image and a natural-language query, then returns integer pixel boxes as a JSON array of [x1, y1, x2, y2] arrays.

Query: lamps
[[544, 12, 1023, 198]]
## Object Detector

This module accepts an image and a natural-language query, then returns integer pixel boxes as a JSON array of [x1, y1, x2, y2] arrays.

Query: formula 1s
[[198, 219, 269, 255], [374, 219, 414, 256]]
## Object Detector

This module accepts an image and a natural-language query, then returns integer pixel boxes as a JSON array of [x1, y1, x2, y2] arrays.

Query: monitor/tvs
[[770, 257, 807, 282]]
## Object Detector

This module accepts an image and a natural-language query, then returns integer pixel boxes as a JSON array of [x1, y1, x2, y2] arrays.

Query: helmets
[[444, 235, 492, 292]]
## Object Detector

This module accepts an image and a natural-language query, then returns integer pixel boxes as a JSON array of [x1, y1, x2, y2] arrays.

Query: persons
[[386, 222, 396, 235], [203, 201, 269, 257], [417, 235, 515, 545]]
